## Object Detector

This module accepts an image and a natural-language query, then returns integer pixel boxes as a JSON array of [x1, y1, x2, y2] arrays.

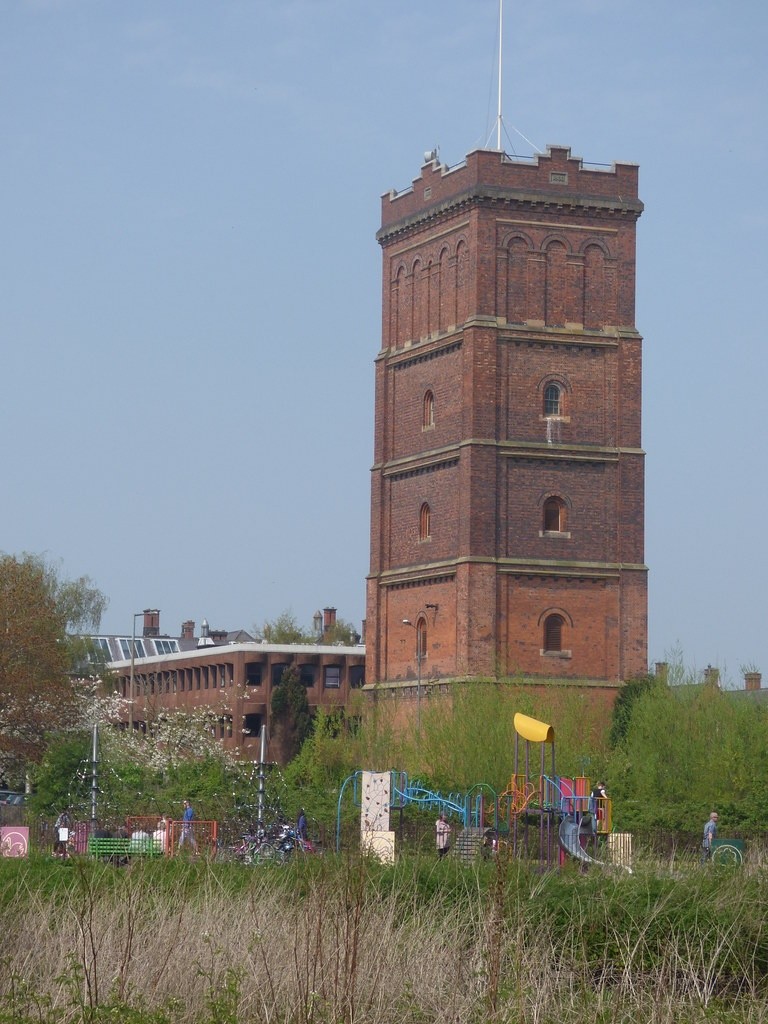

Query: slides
[[557, 812, 636, 874]]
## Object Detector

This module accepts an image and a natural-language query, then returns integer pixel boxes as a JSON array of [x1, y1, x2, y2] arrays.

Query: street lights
[[129, 613, 144, 733], [402, 619, 421, 728]]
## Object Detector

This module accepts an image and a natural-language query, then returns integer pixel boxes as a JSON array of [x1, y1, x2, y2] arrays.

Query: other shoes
[[51, 851, 57, 857], [60, 853, 69, 857]]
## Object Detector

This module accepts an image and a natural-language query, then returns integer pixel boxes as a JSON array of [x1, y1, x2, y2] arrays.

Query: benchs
[[89, 838, 163, 862]]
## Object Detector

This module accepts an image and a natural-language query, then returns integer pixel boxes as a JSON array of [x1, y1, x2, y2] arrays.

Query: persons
[[175, 799, 200, 858], [591, 782, 609, 828], [51, 810, 72, 856], [297, 810, 308, 842], [436, 811, 451, 862], [93, 813, 172, 863], [703, 812, 718, 862], [278, 824, 293, 864]]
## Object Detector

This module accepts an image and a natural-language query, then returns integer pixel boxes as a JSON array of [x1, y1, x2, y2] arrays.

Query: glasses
[[184, 802, 187, 805]]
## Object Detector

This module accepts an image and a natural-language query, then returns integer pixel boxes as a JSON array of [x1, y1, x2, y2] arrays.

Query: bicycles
[[228, 829, 276, 865]]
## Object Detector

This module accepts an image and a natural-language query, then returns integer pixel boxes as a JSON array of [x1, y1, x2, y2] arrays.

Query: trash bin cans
[[710, 839, 745, 882]]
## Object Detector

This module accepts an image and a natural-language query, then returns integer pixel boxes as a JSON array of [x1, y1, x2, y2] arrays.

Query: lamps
[[425, 603, 438, 611]]
